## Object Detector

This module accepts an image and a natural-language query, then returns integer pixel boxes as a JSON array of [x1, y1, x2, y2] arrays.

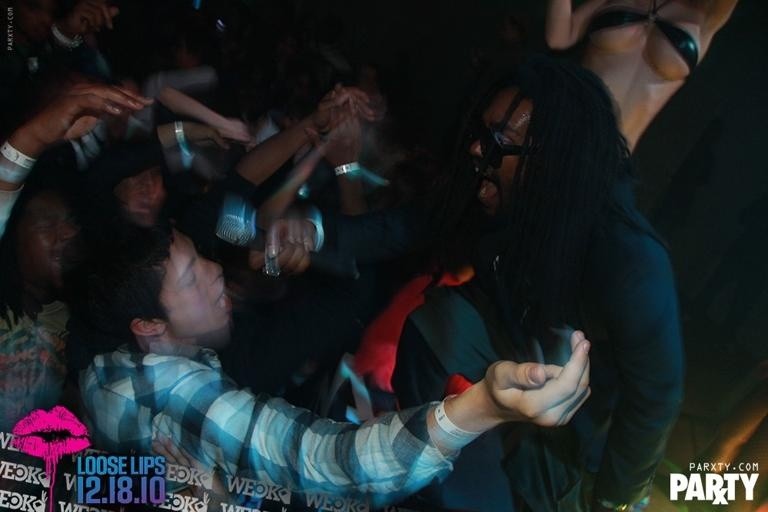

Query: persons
[[1, 0, 767, 511]]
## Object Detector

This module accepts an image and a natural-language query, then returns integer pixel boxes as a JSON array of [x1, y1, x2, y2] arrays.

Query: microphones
[[216, 213, 357, 281]]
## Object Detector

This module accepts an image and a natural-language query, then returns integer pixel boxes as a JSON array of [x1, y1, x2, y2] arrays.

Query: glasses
[[464, 115, 527, 167]]
